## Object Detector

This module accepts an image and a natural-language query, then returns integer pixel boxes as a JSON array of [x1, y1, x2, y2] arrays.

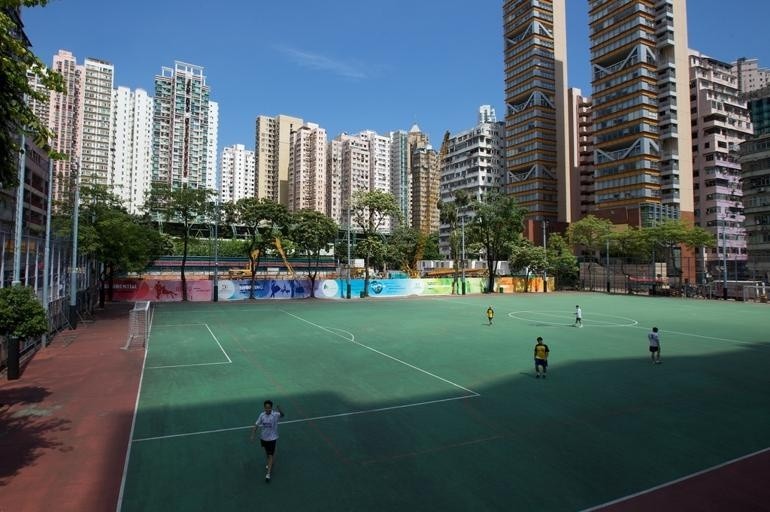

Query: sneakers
[[265, 473, 272, 480]]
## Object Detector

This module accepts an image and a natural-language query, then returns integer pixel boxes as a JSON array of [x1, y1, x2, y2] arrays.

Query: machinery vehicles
[[350, 267, 366, 278], [402, 131, 487, 278], [228, 236, 295, 278]]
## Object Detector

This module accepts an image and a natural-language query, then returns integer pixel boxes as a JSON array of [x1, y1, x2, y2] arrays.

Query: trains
[[151, 218, 390, 244]]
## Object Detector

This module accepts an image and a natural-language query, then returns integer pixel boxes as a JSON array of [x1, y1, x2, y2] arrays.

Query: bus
[[623, 275, 668, 290], [704, 280, 765, 301]]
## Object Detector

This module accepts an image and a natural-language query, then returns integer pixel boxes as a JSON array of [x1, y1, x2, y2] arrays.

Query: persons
[[486, 307, 494, 323], [254, 400, 284, 480], [154, 281, 177, 299], [647, 327, 662, 363], [572, 305, 584, 328], [534, 338, 549, 378]]
